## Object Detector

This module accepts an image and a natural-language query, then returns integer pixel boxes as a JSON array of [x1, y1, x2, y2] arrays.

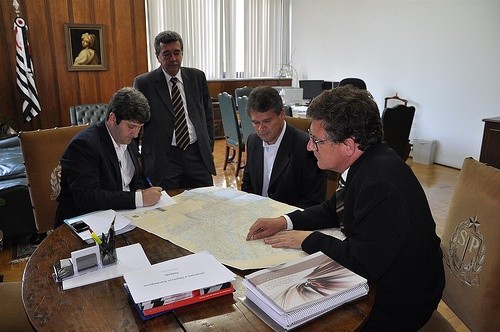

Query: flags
[[15, 18, 40, 124]]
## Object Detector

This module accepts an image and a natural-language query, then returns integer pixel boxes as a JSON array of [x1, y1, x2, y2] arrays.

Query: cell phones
[[70, 220, 90, 232]]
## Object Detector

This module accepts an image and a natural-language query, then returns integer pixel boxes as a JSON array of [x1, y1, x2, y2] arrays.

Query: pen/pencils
[[87, 215, 117, 261], [146, 177, 154, 187]]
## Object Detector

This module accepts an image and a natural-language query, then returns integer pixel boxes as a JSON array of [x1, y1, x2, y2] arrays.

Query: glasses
[[156, 50, 183, 58], [308, 128, 329, 152]]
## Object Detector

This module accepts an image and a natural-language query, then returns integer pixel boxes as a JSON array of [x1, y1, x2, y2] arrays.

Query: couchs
[[0, 136, 38, 239]]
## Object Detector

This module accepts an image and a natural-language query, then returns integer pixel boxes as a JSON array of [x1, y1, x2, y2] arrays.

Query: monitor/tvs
[[299, 80, 324, 99]]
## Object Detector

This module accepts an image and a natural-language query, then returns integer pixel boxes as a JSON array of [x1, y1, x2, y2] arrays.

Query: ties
[[169, 78, 190, 151], [335, 177, 347, 238]]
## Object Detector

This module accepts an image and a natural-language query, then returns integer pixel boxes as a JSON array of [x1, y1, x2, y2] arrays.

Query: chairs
[[18, 125, 91, 241], [379, 105, 415, 163], [419, 156, 500, 332], [217, 86, 254, 177], [68, 103, 109, 125]]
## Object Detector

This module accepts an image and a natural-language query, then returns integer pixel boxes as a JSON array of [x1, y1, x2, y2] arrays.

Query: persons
[[246, 88, 448, 332], [54, 85, 162, 233], [240, 87, 327, 208], [134, 30, 217, 190]]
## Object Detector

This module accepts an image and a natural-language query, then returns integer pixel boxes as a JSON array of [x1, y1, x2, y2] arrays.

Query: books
[[140, 282, 235, 319], [243, 249, 372, 331]]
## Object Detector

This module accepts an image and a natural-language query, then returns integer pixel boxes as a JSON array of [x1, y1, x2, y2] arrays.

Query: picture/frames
[[63, 23, 109, 72]]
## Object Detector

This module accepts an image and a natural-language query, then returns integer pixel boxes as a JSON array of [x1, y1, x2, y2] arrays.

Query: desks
[[22, 188, 377, 332]]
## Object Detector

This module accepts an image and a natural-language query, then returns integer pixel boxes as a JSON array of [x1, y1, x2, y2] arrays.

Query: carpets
[[11, 241, 44, 263]]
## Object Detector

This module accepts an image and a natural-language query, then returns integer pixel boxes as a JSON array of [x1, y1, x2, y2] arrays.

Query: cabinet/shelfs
[[209, 76, 292, 139], [479, 118, 500, 169]]
